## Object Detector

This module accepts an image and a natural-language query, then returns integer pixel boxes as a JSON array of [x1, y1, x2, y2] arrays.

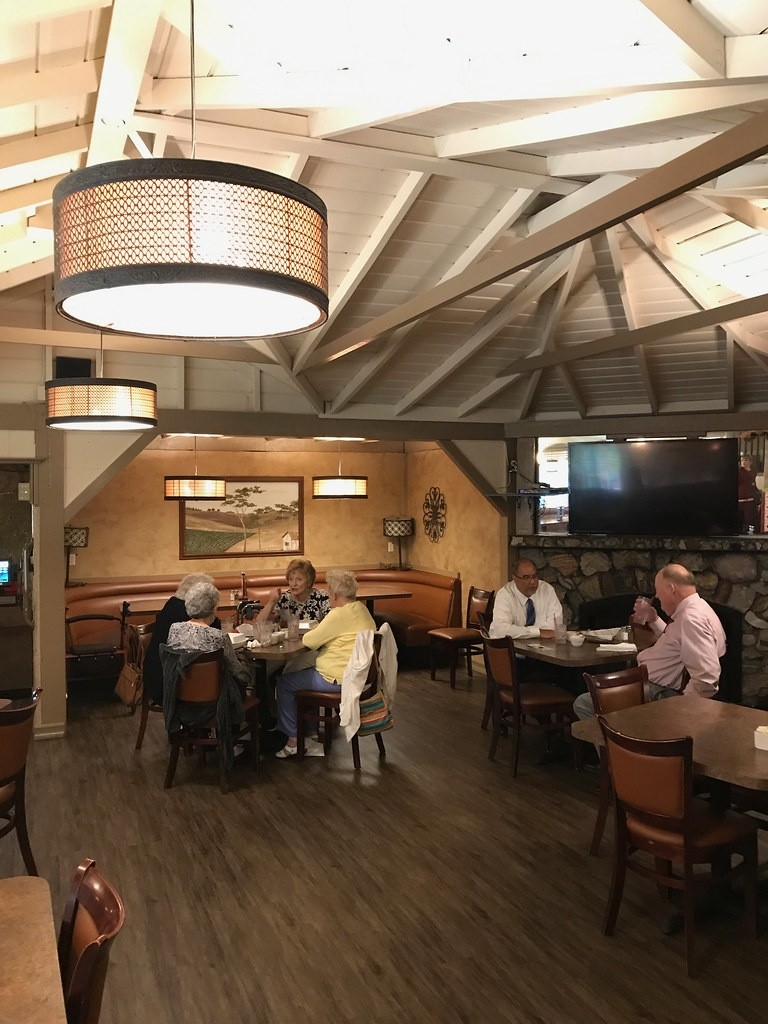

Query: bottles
[[230, 589, 240, 600], [620, 625, 634, 642]]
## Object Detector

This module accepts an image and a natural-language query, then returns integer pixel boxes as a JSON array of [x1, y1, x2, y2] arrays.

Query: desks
[[565, 692, 768, 939], [511, 632, 642, 699], [354, 584, 415, 620], [235, 630, 314, 735], [119, 595, 261, 634]]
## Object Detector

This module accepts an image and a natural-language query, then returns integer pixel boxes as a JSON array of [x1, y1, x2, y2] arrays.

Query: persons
[[140, 571, 254, 763], [572, 563, 727, 795], [254, 559, 331, 701], [488, 558, 564, 729], [274, 568, 377, 760]]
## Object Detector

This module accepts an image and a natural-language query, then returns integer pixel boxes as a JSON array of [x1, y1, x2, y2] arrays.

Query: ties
[[525, 599, 536, 627]]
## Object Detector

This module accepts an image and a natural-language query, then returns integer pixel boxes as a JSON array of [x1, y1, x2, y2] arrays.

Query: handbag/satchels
[[114, 631, 143, 706]]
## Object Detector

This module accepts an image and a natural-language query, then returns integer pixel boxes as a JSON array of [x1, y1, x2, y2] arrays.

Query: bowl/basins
[[270, 631, 285, 645], [232, 638, 249, 650], [570, 635, 585, 647], [566, 631, 578, 641]]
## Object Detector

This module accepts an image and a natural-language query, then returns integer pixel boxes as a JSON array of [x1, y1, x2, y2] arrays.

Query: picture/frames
[[178, 475, 305, 561]]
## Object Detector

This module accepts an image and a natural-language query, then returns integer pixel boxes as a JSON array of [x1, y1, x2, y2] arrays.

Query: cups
[[555, 624, 567, 644], [253, 619, 273, 647], [287, 615, 300, 641], [633, 596, 652, 625]]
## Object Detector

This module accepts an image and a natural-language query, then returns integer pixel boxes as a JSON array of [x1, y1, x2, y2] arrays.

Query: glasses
[[514, 574, 538, 581]]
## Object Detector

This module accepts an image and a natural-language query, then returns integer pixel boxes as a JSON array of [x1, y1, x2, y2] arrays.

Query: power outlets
[[387, 541, 395, 553], [69, 553, 76, 566]]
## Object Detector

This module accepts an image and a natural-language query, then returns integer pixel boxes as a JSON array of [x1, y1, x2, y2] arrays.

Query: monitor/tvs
[[568, 438, 740, 536]]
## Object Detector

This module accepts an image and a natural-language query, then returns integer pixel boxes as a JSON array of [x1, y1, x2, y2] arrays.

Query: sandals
[[310, 734, 319, 742], [276, 746, 298, 757]]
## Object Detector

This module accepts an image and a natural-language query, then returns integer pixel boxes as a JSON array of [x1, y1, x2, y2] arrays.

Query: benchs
[[64, 566, 462, 671]]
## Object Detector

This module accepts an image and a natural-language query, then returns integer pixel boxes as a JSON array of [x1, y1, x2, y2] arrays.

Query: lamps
[[43, 329, 160, 432], [49, 0, 332, 343], [382, 515, 416, 572], [163, 432, 227, 503], [311, 437, 370, 502], [63, 524, 90, 589]]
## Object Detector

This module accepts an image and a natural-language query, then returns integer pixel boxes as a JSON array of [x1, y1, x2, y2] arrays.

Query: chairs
[[64, 599, 388, 796], [56, 856, 127, 1024], [0, 686, 44, 879], [425, 584, 760, 982]]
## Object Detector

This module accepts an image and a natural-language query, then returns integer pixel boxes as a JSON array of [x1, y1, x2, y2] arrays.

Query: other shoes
[[498, 724, 508, 736], [233, 743, 246, 758], [267, 717, 278, 732], [584, 756, 601, 773], [535, 750, 553, 764]]
